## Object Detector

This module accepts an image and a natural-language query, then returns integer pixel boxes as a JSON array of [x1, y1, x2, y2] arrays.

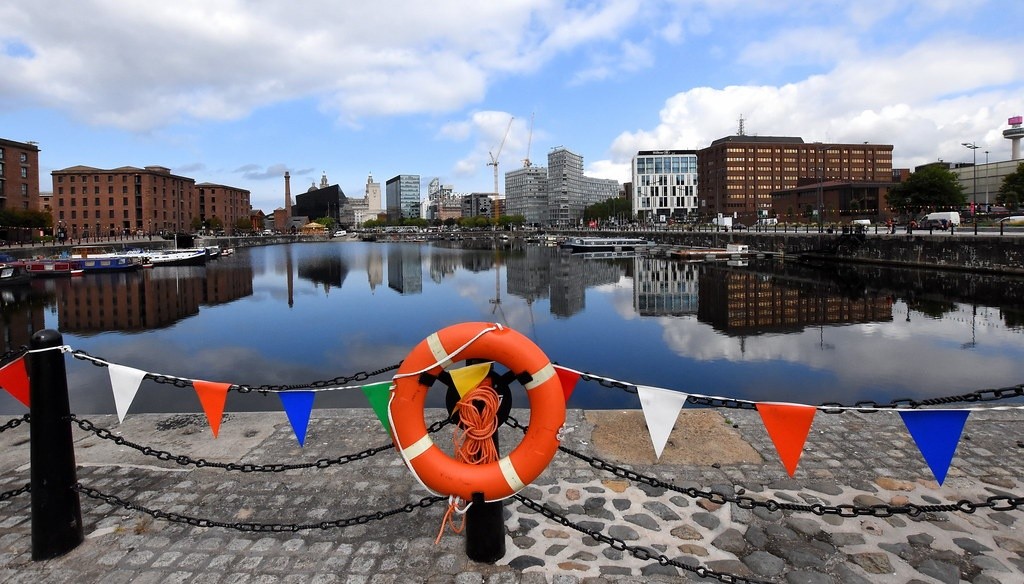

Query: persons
[[666, 218, 672, 228], [625, 218, 629, 226], [885, 215, 897, 234], [631, 218, 637, 227]]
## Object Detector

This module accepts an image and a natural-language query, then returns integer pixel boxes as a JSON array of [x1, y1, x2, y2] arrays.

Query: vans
[[848, 220, 870, 225], [917, 212, 960, 230], [996, 216, 1024, 226], [333, 230, 347, 237], [755, 218, 778, 226]]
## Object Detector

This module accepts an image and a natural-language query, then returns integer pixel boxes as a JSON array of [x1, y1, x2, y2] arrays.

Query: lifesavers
[[383, 319, 569, 504]]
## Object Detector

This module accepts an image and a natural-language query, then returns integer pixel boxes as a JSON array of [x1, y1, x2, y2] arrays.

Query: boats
[[0, 253, 31, 284], [453, 233, 769, 260], [33, 245, 143, 270], [0, 259, 85, 275], [105, 233, 234, 264]]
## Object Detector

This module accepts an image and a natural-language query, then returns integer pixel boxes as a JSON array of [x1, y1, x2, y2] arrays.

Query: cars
[[733, 223, 746, 230], [917, 219, 949, 230]]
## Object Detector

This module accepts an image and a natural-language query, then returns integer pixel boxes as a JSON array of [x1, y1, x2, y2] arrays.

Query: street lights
[[961, 142, 981, 236]]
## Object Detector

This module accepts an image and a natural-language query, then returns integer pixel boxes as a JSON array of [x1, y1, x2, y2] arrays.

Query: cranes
[[485, 115, 516, 224], [519, 109, 536, 169]]
